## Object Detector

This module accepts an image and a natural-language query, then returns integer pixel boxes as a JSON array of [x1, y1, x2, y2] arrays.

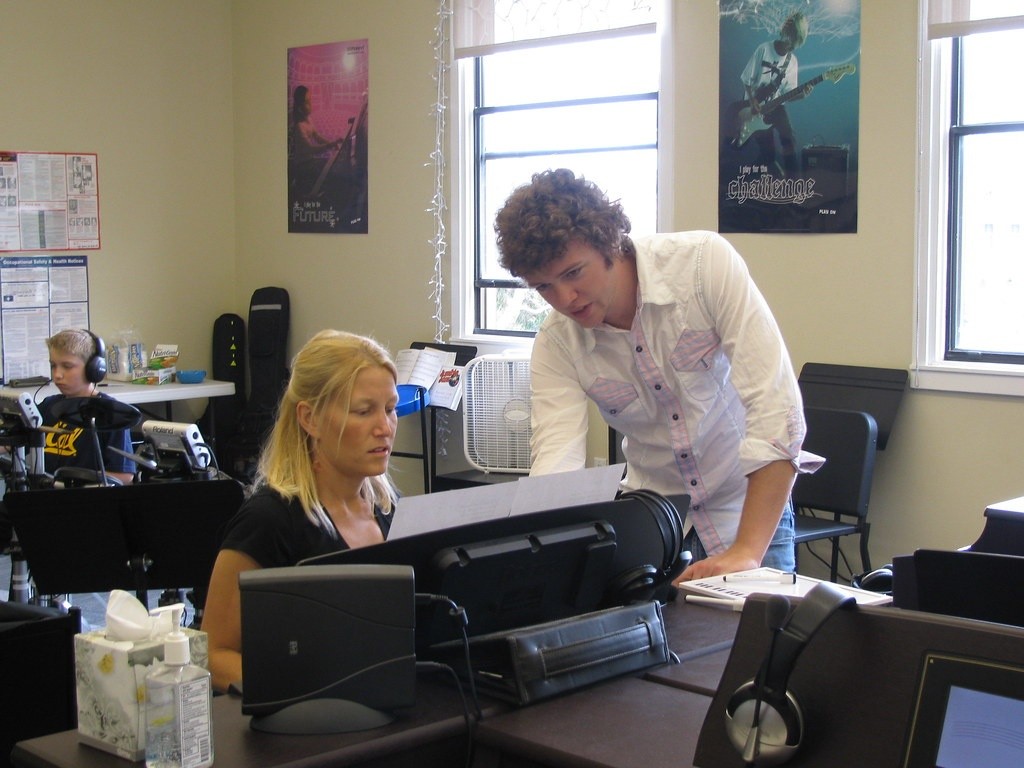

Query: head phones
[[81, 329, 106, 381]]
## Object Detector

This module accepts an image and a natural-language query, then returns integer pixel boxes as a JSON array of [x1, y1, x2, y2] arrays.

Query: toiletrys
[[140, 601, 214, 768]]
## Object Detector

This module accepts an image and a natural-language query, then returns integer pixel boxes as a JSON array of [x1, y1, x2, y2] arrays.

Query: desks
[[0, 373, 236, 422]]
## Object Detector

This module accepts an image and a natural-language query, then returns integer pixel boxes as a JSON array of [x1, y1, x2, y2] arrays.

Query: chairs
[[792, 404, 878, 583]]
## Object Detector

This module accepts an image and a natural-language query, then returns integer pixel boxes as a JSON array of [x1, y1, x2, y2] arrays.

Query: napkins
[[75, 587, 213, 762]]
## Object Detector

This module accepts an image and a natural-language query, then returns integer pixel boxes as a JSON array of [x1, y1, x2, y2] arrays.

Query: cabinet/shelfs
[[1, 495, 1024, 767], [436, 467, 528, 493]]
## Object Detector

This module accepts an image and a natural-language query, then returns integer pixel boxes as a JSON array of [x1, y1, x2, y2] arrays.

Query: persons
[[293, 84, 342, 187], [199, 329, 405, 693], [739, 13, 813, 189], [0, 328, 138, 488], [491, 168, 827, 583]]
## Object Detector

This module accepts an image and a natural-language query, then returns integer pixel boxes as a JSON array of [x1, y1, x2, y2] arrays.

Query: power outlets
[[593, 457, 607, 467]]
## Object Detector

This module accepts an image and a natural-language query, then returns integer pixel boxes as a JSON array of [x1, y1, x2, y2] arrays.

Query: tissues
[[70, 587, 212, 764]]
